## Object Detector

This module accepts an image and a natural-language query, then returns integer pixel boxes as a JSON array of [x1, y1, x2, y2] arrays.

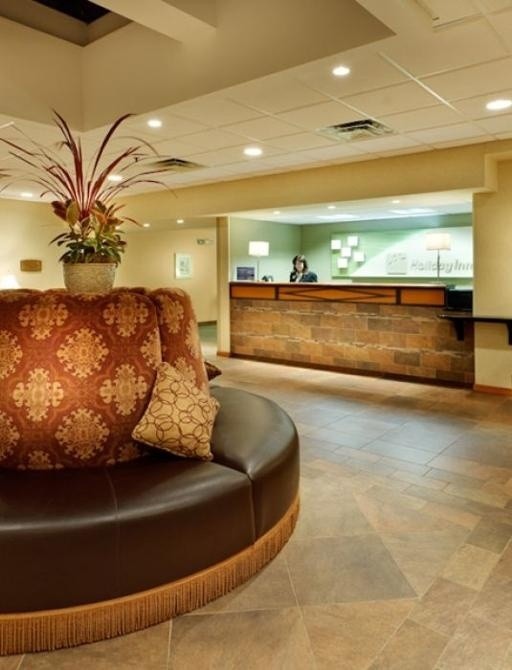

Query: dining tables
[[1, 100, 208, 300]]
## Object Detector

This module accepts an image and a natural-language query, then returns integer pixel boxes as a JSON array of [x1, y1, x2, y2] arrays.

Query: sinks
[[0, 289, 304, 659]]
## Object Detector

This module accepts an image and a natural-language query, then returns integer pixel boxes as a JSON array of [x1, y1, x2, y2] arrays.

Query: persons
[[290, 255, 317, 282]]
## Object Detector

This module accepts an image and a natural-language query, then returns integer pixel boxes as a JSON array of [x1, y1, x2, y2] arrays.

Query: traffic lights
[[132, 360, 222, 464]]
